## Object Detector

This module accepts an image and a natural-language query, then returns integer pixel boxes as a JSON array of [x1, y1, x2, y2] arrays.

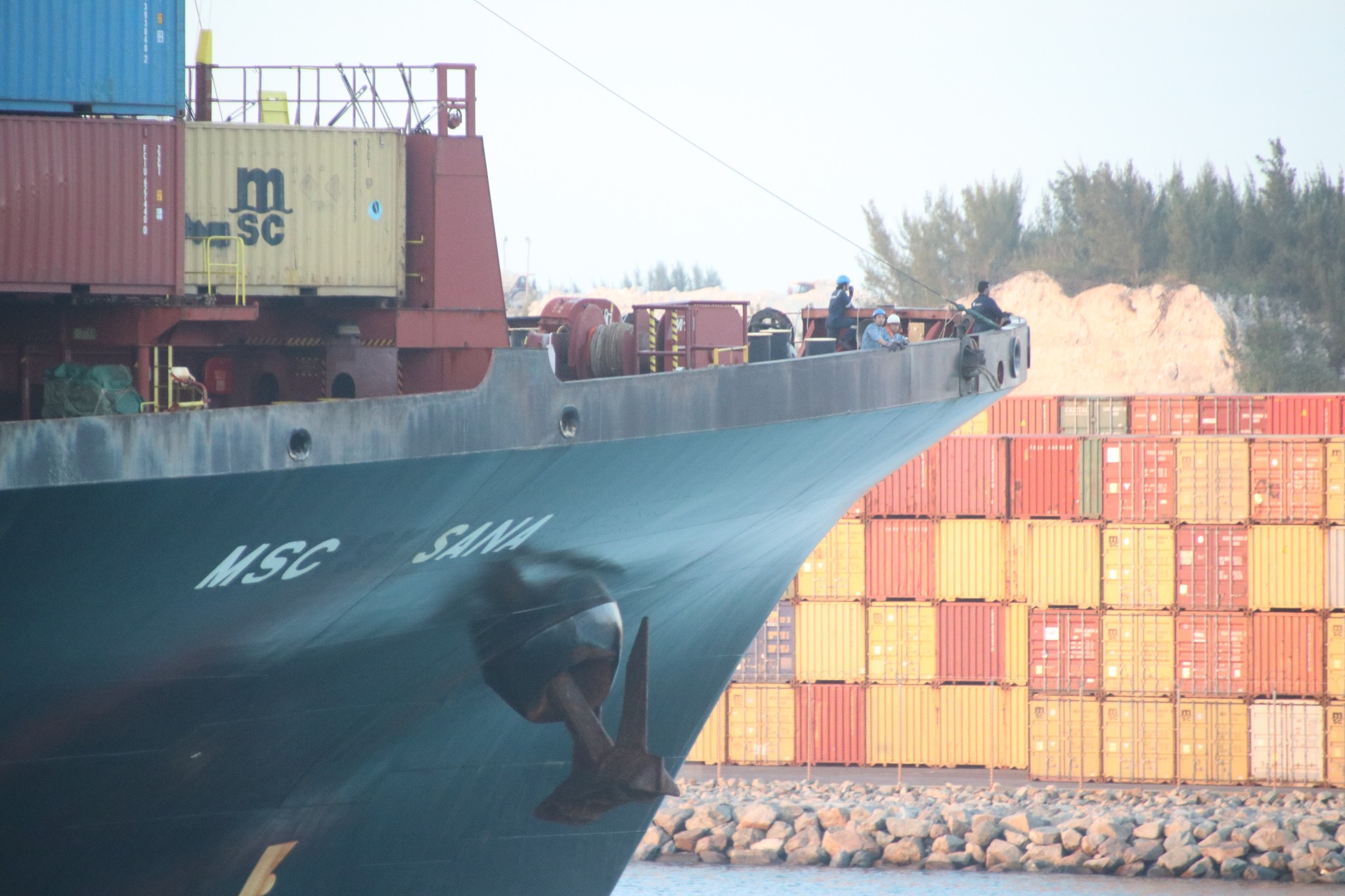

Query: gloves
[[887, 343, 897, 352], [902, 339, 910, 345], [897, 343, 905, 351]]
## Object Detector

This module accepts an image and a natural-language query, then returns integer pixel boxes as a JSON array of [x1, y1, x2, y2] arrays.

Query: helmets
[[837, 275, 850, 284], [887, 314, 901, 325], [873, 309, 886, 316]]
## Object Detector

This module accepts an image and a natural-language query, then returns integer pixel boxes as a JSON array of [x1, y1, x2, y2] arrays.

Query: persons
[[825, 274, 858, 349], [883, 313, 909, 345], [971, 281, 1002, 334], [860, 308, 905, 352]]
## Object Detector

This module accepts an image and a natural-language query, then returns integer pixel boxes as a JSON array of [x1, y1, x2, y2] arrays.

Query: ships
[[0, 31, 1029, 895]]
